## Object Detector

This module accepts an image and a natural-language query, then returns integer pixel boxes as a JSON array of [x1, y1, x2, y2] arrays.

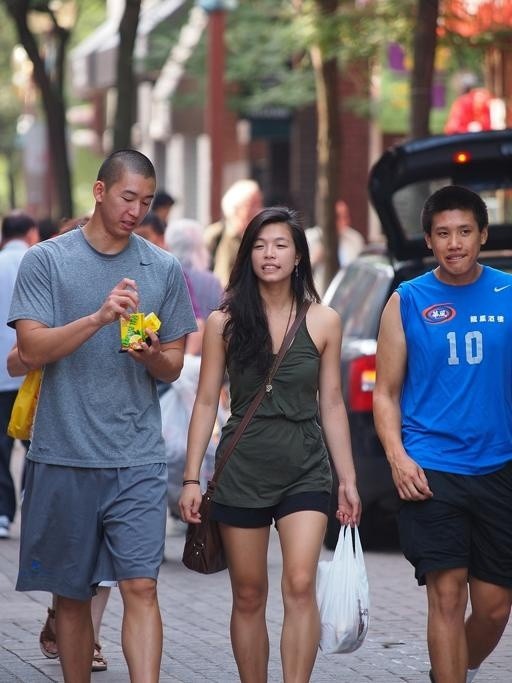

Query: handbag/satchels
[[6, 368, 44, 440], [183, 482, 228, 573]]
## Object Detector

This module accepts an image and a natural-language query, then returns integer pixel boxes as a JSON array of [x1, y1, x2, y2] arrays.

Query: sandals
[[40, 607, 58, 658], [91, 642, 108, 671]]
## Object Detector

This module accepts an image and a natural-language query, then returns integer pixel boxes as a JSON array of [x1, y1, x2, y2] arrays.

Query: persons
[[176, 207, 365, 683], [375, 186, 512, 679], [3, 151, 202, 681], [2, 179, 365, 672]]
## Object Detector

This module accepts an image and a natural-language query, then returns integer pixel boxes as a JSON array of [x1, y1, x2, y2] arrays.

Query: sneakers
[[0, 514, 11, 538]]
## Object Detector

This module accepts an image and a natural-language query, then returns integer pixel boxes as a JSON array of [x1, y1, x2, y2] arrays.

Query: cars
[[310, 128, 511, 551]]
